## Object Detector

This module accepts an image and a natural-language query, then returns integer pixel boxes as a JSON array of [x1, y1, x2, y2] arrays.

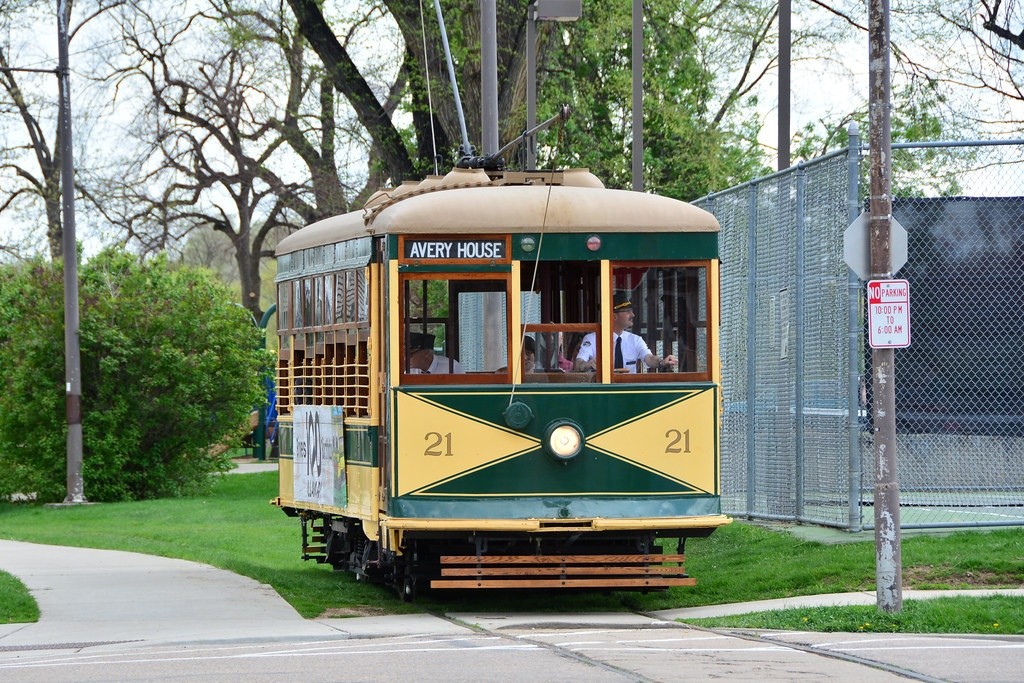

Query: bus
[[269, 0, 733, 605]]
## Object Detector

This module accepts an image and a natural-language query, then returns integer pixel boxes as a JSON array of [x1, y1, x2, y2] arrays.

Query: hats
[[410, 332, 436, 350], [597, 293, 637, 312]]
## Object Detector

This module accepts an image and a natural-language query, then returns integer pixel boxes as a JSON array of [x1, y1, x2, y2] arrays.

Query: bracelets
[[659, 359, 664, 368]]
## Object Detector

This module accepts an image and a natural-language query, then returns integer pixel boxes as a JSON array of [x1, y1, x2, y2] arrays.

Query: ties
[[615, 337, 623, 368]]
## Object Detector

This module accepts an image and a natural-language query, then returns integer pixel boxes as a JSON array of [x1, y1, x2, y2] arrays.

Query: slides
[[183, 409, 260, 462]]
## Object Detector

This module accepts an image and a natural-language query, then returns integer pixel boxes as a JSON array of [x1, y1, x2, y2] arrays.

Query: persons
[[495, 322, 574, 374], [574, 292, 676, 372], [404, 331, 466, 374]]
